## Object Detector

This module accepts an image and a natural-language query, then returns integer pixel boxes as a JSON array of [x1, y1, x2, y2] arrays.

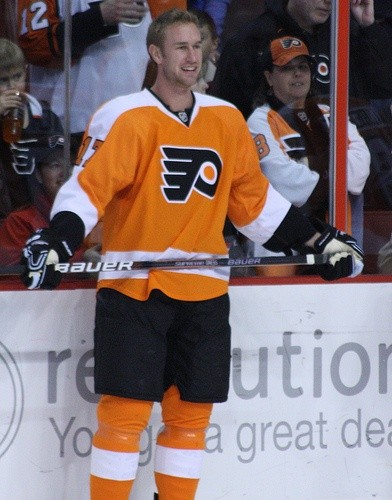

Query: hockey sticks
[[54, 250, 344, 272]]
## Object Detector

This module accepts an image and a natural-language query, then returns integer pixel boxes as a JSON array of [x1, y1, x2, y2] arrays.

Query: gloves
[[306, 216, 366, 279], [20, 227, 75, 289]]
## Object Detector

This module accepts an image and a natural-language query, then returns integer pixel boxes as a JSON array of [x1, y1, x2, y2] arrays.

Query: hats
[[261, 37, 317, 68], [28, 131, 80, 168]]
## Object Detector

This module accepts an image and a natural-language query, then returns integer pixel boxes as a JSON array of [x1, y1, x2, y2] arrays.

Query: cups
[[2, 89, 24, 143]]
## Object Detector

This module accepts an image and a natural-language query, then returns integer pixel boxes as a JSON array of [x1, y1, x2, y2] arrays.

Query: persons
[[0, 135, 102, 278], [0, 38, 66, 222], [22, 7, 364, 500], [246, 37, 370, 276], [0, 0, 392, 280]]
